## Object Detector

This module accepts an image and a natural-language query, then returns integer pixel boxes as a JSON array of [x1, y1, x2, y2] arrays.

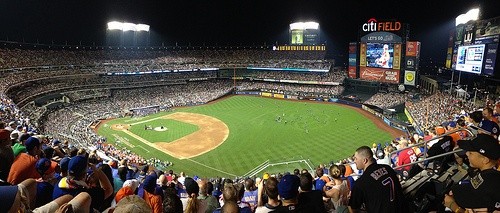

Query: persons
[[0, 40, 500, 213]]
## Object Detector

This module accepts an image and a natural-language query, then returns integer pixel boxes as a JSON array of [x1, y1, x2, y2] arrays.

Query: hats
[[434, 121, 456, 134], [278, 175, 300, 198], [35, 158, 58, 173], [115, 187, 132, 204], [122, 179, 138, 192], [470, 119, 500, 137], [53, 140, 61, 144], [25, 135, 42, 147], [60, 157, 71, 170], [452, 168, 500, 209], [186, 179, 199, 194], [43, 147, 54, 155], [68, 156, 87, 173], [143, 173, 157, 190], [457, 133, 500, 160]]
